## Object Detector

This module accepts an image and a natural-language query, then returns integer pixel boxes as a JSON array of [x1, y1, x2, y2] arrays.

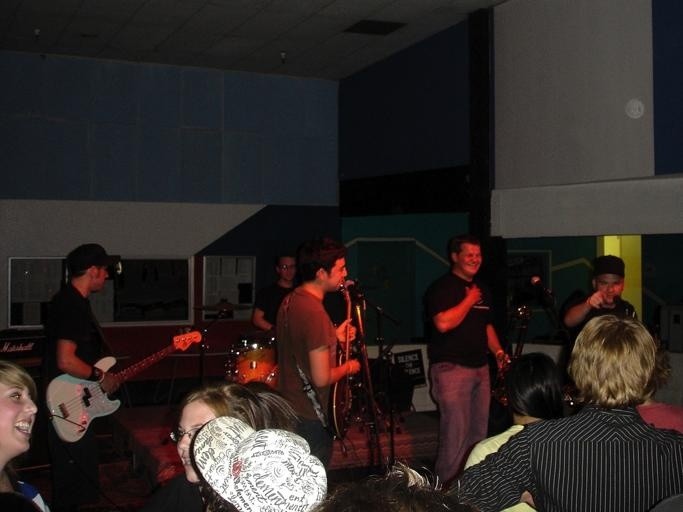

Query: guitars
[[327, 284, 354, 440], [47, 332, 203, 443]]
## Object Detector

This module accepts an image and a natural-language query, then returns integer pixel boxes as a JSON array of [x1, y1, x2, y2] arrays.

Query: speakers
[[0, 328, 56, 469]]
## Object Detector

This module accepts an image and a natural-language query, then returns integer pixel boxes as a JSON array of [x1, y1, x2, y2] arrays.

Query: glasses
[[169, 429, 196, 442]]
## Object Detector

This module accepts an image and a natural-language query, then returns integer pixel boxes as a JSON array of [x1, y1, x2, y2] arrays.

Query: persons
[[39, 244, 121, 512], [0, 360, 51, 512]]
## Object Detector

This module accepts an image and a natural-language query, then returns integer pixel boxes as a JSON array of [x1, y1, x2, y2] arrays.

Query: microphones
[[531, 276, 555, 302], [613, 295, 634, 311], [344, 280, 365, 301]]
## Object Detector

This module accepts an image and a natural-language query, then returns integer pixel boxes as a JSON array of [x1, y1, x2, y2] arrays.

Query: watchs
[[87, 366, 100, 383]]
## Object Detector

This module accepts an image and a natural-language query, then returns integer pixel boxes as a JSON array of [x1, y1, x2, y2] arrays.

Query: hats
[[593, 256, 624, 278], [66, 244, 122, 272], [193, 416, 328, 512]]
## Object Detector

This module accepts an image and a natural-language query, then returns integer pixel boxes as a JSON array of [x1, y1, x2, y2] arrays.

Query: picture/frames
[[87, 254, 195, 329]]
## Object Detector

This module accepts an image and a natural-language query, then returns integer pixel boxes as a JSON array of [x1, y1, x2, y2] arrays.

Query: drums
[[229, 337, 279, 389]]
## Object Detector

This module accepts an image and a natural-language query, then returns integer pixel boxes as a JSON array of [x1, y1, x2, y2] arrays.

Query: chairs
[[113, 353, 136, 407]]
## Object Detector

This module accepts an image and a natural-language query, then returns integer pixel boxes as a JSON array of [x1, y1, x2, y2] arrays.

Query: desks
[[162, 349, 237, 406]]
